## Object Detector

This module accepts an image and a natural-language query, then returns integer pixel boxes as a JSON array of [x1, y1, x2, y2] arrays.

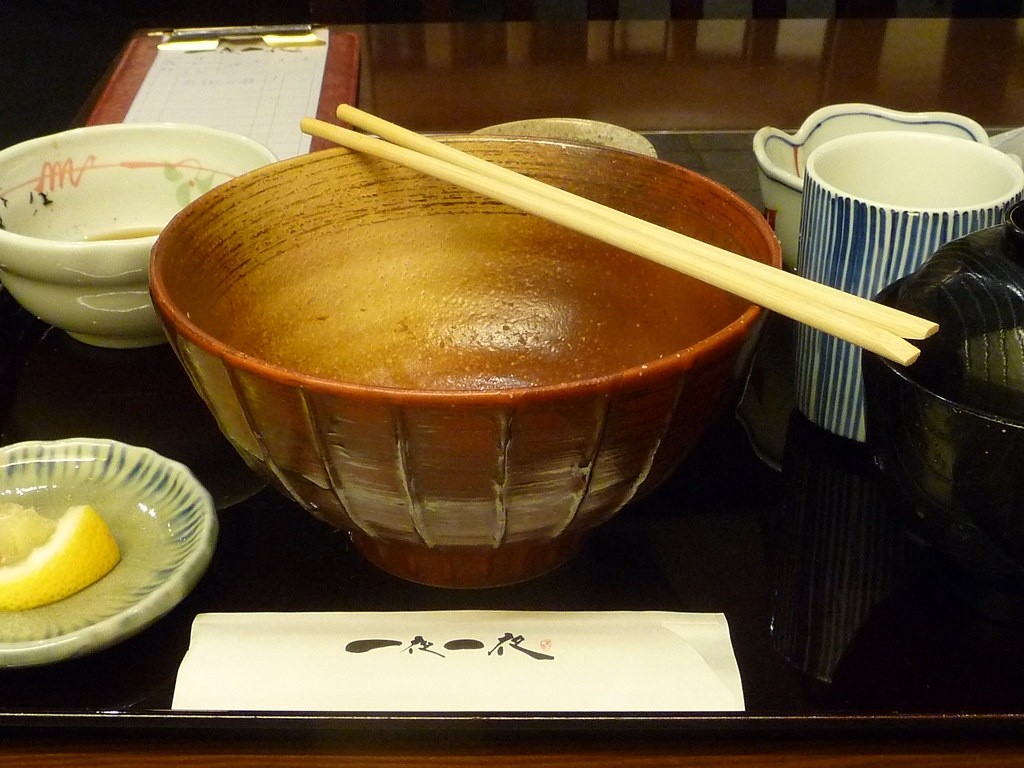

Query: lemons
[[0, 500, 122, 612]]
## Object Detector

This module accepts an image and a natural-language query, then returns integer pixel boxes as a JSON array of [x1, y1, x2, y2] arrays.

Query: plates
[[0, 437, 219, 668]]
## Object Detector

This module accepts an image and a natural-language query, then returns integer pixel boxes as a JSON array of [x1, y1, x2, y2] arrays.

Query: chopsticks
[[299, 103, 939, 365]]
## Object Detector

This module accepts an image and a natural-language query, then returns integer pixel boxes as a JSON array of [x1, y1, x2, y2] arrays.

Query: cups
[[792, 130, 1024, 442]]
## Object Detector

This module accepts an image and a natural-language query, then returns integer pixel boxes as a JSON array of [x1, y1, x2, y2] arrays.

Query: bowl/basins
[[147, 132, 784, 589], [469, 118, 659, 160], [753, 104, 1024, 273], [867, 200, 1024, 638], [0, 124, 280, 349]]
